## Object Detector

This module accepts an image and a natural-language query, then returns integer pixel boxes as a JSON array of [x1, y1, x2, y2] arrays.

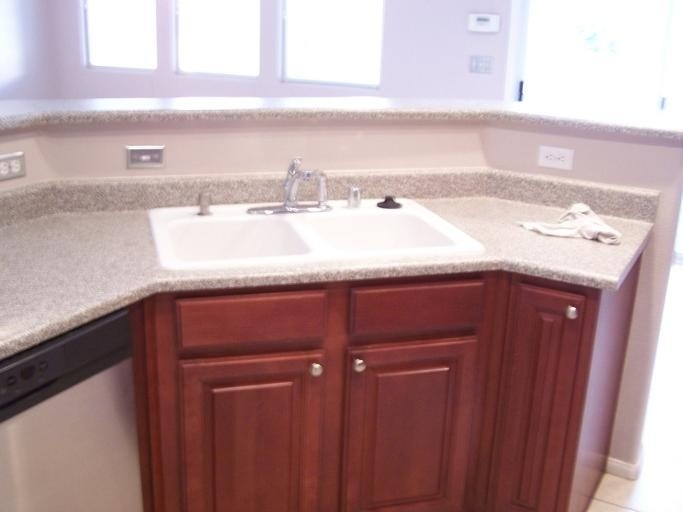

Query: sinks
[[147, 201, 484, 271]]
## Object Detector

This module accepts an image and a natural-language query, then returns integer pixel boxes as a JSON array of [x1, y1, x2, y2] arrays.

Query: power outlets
[[0, 151, 27, 182], [536, 145, 574, 171]]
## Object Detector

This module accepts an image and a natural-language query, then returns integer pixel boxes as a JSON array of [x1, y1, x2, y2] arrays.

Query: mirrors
[[1, 0, 680, 132]]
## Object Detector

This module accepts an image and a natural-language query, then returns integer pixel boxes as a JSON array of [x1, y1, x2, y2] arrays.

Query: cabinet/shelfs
[[484, 250, 642, 511], [128, 269, 499, 512]]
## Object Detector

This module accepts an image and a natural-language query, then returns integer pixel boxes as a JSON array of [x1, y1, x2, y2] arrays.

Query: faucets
[[282, 157, 312, 205]]
